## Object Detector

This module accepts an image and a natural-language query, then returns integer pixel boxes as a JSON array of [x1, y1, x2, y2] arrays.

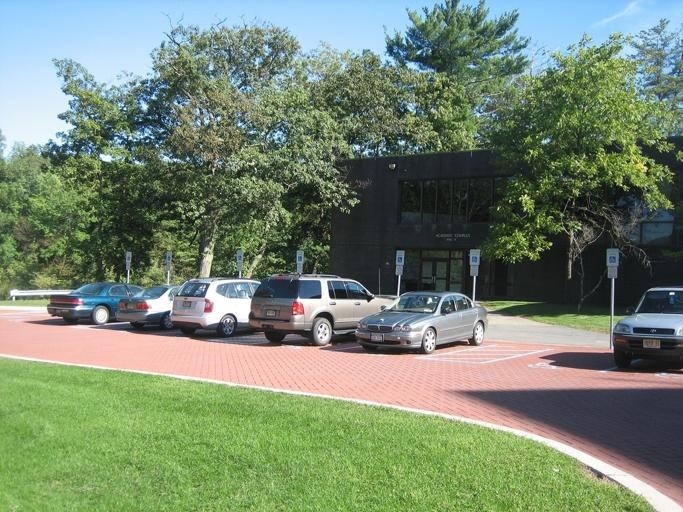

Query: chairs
[[411, 296, 467, 311]]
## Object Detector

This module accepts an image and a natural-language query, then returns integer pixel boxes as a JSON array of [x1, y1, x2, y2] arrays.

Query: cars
[[613, 286, 683, 368]]
